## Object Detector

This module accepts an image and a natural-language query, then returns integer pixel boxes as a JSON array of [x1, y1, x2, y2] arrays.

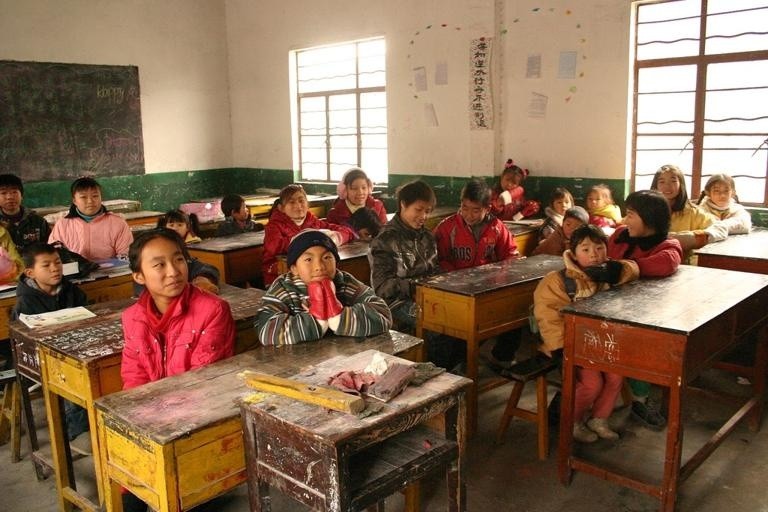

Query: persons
[[0, 224, 26, 284], [47, 177, 135, 265], [9, 242, 88, 460], [0, 173, 52, 358]]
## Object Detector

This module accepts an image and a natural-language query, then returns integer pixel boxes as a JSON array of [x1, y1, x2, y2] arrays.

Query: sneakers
[[572, 418, 598, 443], [629, 397, 666, 432], [587, 417, 620, 441]]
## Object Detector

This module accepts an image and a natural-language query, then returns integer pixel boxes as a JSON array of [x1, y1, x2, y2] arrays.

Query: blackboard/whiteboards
[[0, 60, 146, 185]]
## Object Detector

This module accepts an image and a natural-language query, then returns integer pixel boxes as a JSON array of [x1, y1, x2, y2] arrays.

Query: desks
[[0, 185, 563, 512], [692, 227, 768, 383], [560, 264, 768, 512]]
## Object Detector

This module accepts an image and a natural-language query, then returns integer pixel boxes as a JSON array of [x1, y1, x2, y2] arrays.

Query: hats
[[286, 227, 340, 270]]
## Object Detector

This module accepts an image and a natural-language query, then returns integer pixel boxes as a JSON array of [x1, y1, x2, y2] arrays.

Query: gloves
[[321, 277, 344, 319], [308, 281, 327, 319], [586, 260, 622, 284]]
